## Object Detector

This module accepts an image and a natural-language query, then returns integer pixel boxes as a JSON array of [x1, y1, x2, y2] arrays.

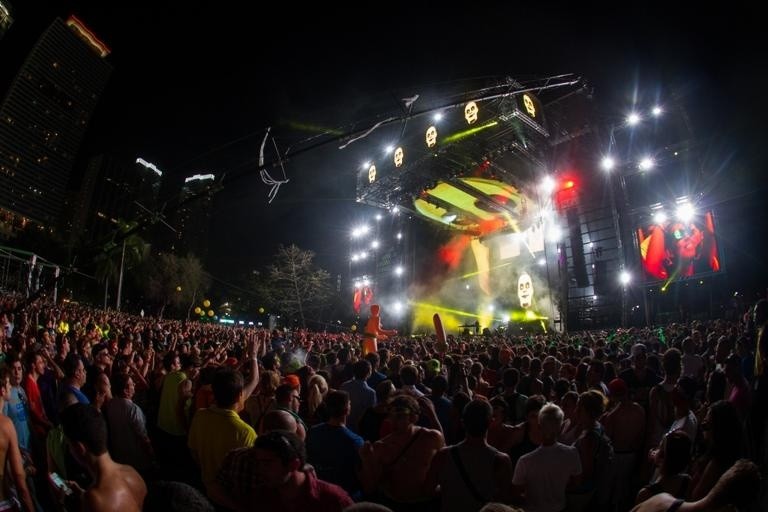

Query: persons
[[517, 272, 534, 309], [464, 101, 479, 125], [0, 281, 767, 512], [523, 94, 536, 118], [393, 147, 403, 168], [663, 223, 705, 261], [425, 126, 437, 148], [368, 165, 376, 183]]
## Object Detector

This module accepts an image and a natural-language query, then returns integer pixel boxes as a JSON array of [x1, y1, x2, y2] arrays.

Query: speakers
[[566, 208, 590, 288]]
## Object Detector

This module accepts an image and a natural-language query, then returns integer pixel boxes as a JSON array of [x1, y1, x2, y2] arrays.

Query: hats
[[427, 359, 441, 373], [284, 375, 300, 385]]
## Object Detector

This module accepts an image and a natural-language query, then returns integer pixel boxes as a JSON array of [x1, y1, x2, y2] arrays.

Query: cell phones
[[48, 470, 72, 496]]
